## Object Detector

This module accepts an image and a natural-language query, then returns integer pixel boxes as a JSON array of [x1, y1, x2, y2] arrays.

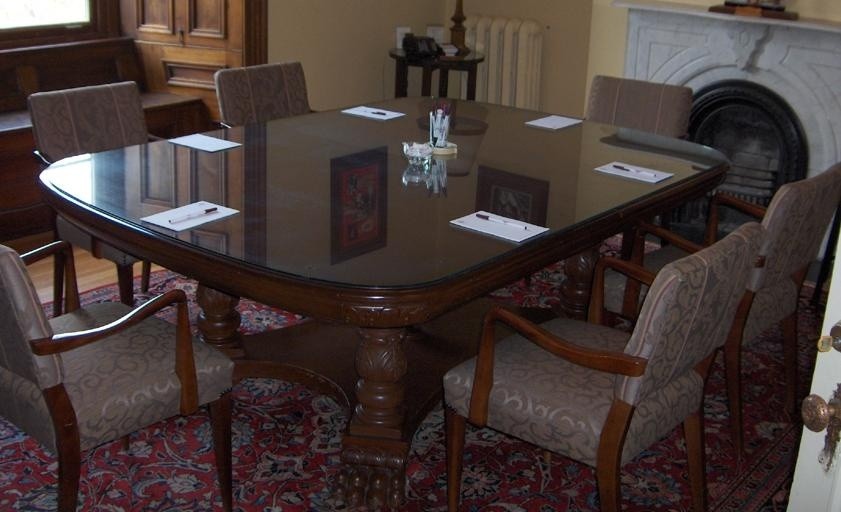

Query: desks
[[383, 45, 485, 100], [36, 90, 740, 511]]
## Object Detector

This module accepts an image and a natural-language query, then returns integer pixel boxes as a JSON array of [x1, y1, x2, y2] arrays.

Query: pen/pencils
[[476, 214, 527, 230], [613, 164, 657, 176], [426, 159, 448, 199], [429, 96, 451, 148], [169, 208, 217, 222], [356, 108, 385, 114]]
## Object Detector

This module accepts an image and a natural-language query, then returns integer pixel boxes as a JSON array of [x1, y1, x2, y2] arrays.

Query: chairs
[[583, 73, 696, 262], [0, 229, 253, 511], [214, 58, 327, 130], [433, 211, 766, 509], [582, 154, 841, 463], [20, 74, 175, 319]]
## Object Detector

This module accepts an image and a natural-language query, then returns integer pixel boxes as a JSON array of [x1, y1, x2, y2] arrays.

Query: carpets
[[0, 232, 832, 511]]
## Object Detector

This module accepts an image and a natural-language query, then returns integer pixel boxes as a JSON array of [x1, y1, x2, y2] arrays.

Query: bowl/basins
[[400, 165, 433, 188], [402, 142, 436, 166]]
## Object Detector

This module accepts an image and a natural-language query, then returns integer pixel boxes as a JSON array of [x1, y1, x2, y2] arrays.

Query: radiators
[[458, 12, 546, 114]]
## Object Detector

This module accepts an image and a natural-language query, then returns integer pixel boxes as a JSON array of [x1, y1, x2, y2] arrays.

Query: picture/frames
[[327, 142, 392, 267], [474, 164, 552, 229]]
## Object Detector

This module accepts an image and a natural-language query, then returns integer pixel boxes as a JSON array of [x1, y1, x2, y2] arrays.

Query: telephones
[[403, 33, 442, 58]]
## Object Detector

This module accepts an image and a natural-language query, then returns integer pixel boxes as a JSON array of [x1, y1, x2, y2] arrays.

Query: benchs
[[2, 34, 208, 243]]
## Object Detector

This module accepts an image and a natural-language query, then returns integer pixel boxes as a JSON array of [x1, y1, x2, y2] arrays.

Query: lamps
[[414, 98, 491, 178]]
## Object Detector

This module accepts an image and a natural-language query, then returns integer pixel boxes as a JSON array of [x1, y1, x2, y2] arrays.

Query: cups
[[424, 157, 449, 190], [427, 111, 451, 147]]
